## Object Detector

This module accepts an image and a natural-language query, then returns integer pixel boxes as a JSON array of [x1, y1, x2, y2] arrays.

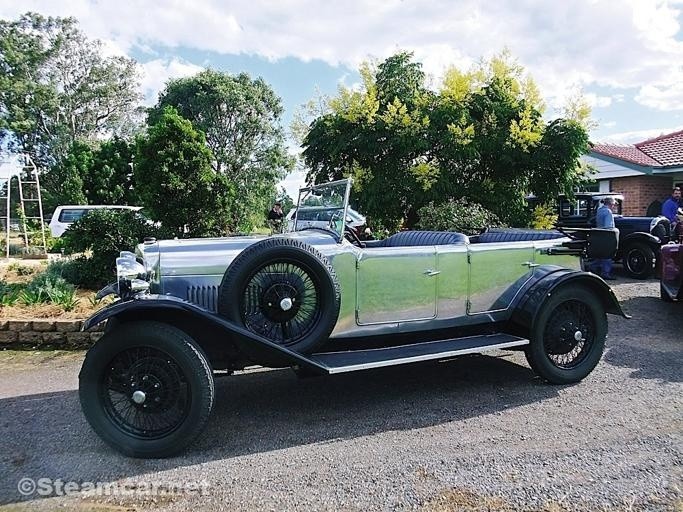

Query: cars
[[285, 206, 389, 240]]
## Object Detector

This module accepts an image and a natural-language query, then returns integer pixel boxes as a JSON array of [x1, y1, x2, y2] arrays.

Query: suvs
[[523, 191, 673, 281], [660, 221, 683, 309], [76, 174, 636, 459]]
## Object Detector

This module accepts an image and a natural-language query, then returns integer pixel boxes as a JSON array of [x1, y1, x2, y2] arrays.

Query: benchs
[[371, 230, 469, 247], [477, 229, 563, 243]]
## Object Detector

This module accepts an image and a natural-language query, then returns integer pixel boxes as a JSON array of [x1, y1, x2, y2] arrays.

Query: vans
[[49, 205, 163, 245]]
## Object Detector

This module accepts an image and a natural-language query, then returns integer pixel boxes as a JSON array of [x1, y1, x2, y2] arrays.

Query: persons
[[268, 202, 284, 228], [660, 187, 683, 229], [594, 196, 616, 279]]
